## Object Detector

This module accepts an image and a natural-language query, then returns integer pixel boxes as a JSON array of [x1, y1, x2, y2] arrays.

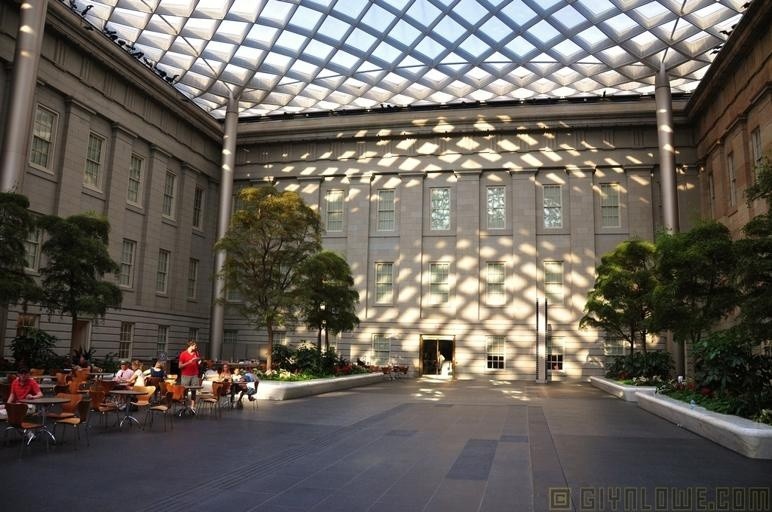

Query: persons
[[0, 339, 259, 440], [438, 351, 445, 375]]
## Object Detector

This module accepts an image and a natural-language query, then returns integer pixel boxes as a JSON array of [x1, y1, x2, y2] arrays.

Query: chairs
[[0, 368, 260, 463]]
